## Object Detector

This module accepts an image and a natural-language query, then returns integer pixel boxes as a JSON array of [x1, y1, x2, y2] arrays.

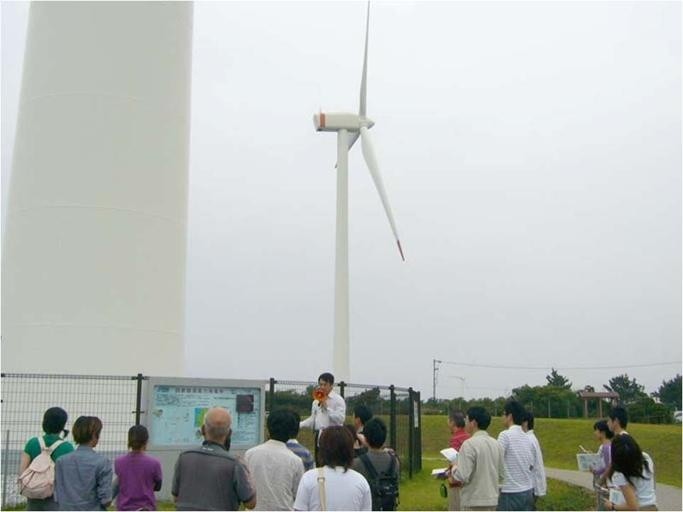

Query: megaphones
[[312, 389, 326, 402]]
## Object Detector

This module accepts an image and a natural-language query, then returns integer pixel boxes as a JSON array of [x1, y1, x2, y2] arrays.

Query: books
[[577, 453, 605, 472]]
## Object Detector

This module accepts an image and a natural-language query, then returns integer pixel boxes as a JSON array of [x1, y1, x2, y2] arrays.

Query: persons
[[115, 425, 162, 511], [590, 407, 657, 511], [440, 401, 546, 511], [299, 373, 346, 467], [53, 415, 113, 510], [19, 408, 77, 511], [170, 406, 401, 511]]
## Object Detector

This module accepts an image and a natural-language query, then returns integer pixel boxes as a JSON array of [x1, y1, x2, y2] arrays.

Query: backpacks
[[18, 436, 67, 501], [359, 451, 401, 510]]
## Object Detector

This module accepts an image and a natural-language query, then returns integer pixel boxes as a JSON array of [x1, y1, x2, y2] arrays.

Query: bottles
[[440, 477, 447, 498]]
